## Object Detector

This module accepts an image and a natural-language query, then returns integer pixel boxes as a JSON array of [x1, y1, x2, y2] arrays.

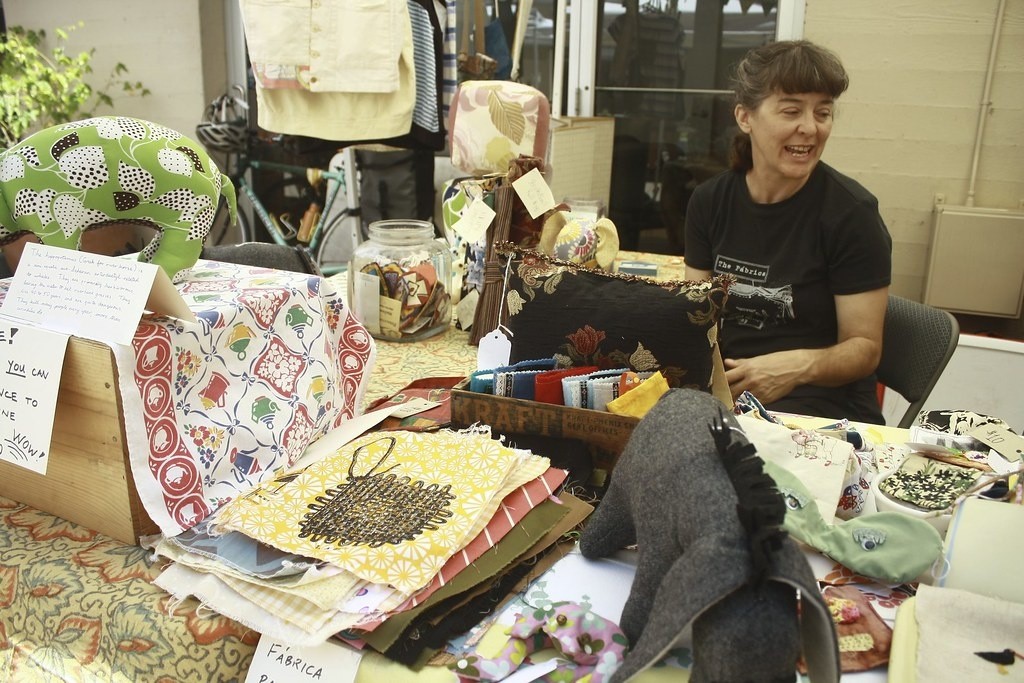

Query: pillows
[[490, 240, 734, 393]]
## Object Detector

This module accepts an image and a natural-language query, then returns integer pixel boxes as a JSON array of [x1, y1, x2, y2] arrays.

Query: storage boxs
[[0, 251, 336, 549]]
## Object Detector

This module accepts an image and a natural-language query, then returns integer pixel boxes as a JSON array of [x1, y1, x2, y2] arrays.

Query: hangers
[[612, 3, 685, 40]]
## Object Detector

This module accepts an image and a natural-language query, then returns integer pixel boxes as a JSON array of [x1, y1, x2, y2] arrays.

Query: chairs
[[870, 290, 961, 429]]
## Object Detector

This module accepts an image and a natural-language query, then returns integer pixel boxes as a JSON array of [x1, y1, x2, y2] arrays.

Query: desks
[[0, 240, 1022, 683]]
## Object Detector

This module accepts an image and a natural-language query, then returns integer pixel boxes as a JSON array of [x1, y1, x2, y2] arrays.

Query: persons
[[682, 40, 897, 427]]
